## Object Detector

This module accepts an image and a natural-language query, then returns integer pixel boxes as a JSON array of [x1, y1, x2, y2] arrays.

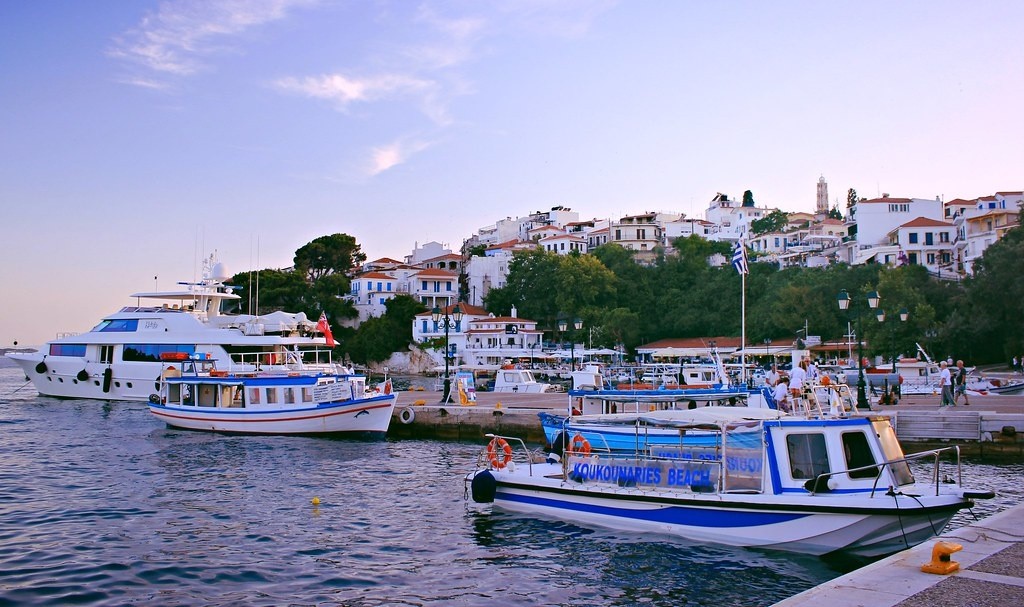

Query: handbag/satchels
[[955, 384, 965, 391]]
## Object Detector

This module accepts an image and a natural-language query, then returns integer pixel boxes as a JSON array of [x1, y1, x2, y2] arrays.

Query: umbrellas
[[511, 347, 628, 369]]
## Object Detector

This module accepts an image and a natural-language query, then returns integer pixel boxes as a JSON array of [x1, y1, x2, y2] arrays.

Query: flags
[[732, 232, 751, 276], [315, 310, 336, 348]]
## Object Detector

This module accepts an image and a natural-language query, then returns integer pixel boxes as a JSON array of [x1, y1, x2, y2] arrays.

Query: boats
[[464, 385, 996, 568], [434, 226, 1024, 463], [4, 223, 349, 402], [146, 352, 398, 442]]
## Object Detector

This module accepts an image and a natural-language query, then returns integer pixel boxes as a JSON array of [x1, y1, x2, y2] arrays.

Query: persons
[[1013, 356, 1024, 376], [765, 356, 847, 416], [938, 356, 970, 407], [572, 407, 581, 415]]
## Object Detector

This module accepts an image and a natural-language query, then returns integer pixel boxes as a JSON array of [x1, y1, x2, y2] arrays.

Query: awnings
[[730, 348, 796, 359], [650, 349, 708, 357]]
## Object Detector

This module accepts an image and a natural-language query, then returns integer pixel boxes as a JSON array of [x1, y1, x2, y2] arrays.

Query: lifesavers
[[384, 381, 391, 394], [265, 351, 276, 365], [487, 438, 512, 469], [399, 406, 415, 424], [567, 436, 591, 458]]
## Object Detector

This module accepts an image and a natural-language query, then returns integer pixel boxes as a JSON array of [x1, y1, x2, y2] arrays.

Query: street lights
[[558, 317, 583, 391], [528, 340, 537, 370], [836, 286, 882, 411], [877, 305, 908, 395], [764, 336, 771, 371], [431, 303, 463, 406]]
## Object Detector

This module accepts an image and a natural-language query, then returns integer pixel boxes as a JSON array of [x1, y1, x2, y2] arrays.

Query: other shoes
[[939, 403, 948, 407], [952, 405, 957, 408], [964, 403, 970, 405]]
